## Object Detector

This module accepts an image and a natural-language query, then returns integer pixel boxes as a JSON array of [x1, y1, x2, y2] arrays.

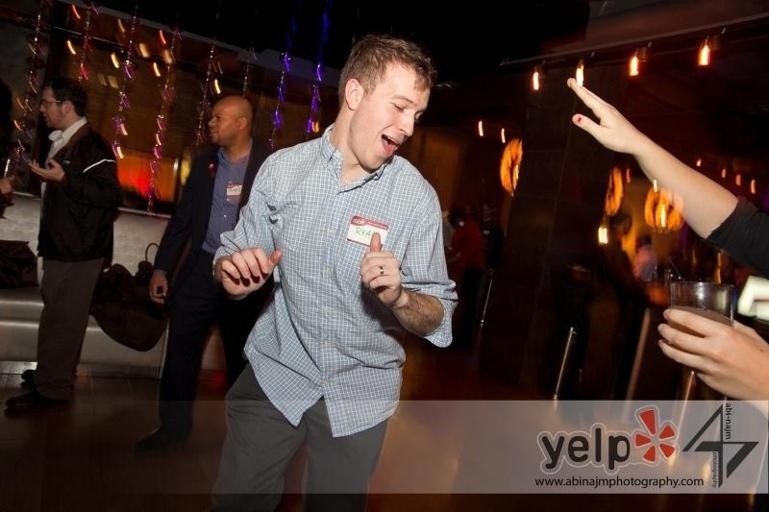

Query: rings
[[379, 265, 385, 276]]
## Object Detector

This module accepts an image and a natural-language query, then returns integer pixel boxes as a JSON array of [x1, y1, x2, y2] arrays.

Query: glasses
[[42, 99, 62, 107]]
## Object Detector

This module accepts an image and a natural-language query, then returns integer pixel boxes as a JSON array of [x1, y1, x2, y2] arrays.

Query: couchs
[[0, 193, 236, 375]]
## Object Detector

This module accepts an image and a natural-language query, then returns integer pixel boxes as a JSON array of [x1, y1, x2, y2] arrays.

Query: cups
[[669, 281, 734, 326]]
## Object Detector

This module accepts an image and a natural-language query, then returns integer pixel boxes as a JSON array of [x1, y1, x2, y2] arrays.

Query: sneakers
[[135, 425, 191, 447], [6, 390, 69, 409], [21, 370, 39, 385]]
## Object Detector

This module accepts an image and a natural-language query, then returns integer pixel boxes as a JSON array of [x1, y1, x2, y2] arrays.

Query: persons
[[563, 73, 768, 510], [478, 201, 505, 282], [578, 209, 656, 399], [0, 78, 20, 221], [125, 91, 270, 459], [445, 207, 487, 349], [4, 72, 124, 416], [195, 32, 464, 511], [632, 234, 662, 286]]
